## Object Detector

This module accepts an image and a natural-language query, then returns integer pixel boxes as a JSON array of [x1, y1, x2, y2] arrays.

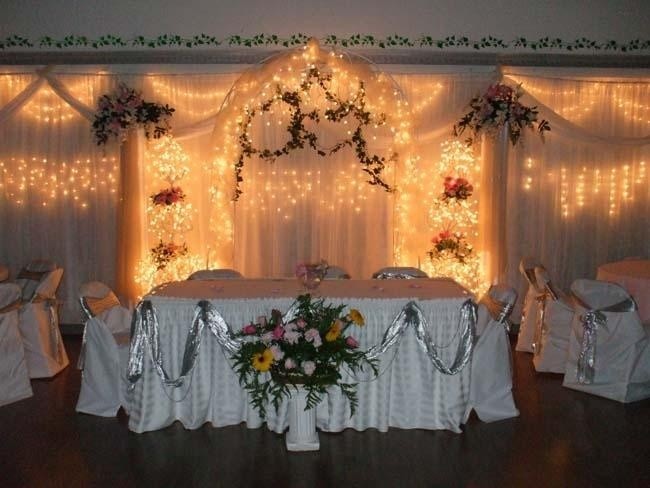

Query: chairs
[[76, 281, 132, 418], [1, 283, 33, 406], [462, 284, 519, 424], [16, 259, 58, 305], [373, 266, 429, 281], [562, 278, 650, 403], [325, 264, 349, 281], [1, 251, 10, 283], [532, 265, 576, 375], [188, 268, 242, 282], [513, 258, 544, 353], [17, 268, 69, 378]]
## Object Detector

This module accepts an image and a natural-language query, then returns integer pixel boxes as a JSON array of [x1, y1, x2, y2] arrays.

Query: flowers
[[424, 81, 551, 266], [75, 83, 189, 268]]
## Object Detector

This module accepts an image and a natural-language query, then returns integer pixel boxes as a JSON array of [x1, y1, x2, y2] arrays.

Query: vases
[[284, 383, 320, 452]]
